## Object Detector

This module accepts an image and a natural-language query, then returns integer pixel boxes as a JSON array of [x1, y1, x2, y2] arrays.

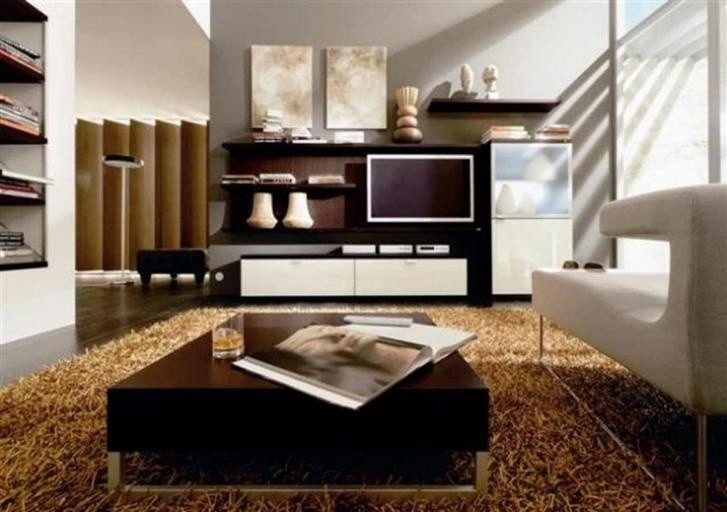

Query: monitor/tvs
[[232, 323, 477, 410], [220, 173, 345, 185], [0, 34, 54, 251], [251, 108, 327, 144], [480, 124, 571, 145]]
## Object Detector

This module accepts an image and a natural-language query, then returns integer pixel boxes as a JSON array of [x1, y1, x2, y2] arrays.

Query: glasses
[[102, 153, 145, 287]]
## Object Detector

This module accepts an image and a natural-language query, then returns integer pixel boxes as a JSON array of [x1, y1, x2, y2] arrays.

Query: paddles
[[0, 304, 727, 512]]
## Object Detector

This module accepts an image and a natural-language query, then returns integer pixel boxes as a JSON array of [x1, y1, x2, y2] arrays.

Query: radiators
[[343, 315, 413, 326]]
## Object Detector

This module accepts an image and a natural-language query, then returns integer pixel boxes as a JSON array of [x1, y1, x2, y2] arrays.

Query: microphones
[[136, 248, 209, 285]]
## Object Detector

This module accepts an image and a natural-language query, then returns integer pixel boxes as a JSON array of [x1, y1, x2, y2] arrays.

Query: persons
[[249, 325, 423, 396]]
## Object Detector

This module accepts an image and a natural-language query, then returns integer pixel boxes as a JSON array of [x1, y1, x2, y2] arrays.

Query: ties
[[367, 155, 474, 223]]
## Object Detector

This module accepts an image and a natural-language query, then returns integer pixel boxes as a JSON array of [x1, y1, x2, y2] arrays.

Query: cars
[[211, 310, 245, 360]]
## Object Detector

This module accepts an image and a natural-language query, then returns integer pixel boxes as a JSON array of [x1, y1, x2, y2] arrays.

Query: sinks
[[531, 182, 726, 512]]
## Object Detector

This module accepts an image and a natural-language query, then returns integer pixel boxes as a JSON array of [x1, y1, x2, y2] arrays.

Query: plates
[[0, 0, 48, 272], [208, 142, 492, 308]]
